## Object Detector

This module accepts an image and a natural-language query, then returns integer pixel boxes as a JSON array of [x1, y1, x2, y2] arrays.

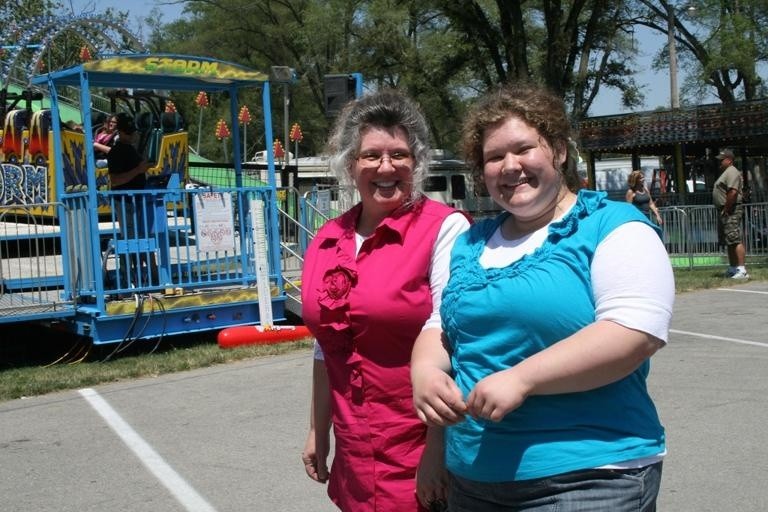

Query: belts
[[717, 203, 740, 211]]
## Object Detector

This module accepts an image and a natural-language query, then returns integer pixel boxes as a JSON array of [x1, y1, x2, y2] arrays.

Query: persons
[[66, 110, 120, 160], [300, 89, 475, 511], [107, 114, 159, 289], [712, 148, 749, 280], [406, 82, 676, 512], [626, 169, 663, 225]]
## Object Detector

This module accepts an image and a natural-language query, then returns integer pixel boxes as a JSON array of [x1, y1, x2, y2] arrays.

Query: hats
[[117, 117, 139, 134], [716, 149, 734, 159]]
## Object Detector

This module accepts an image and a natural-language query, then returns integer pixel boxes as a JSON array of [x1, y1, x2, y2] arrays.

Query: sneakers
[[724, 268, 735, 276], [732, 270, 749, 280]]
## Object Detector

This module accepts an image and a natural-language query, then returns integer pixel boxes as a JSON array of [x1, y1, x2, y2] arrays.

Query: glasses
[[352, 146, 415, 168]]
